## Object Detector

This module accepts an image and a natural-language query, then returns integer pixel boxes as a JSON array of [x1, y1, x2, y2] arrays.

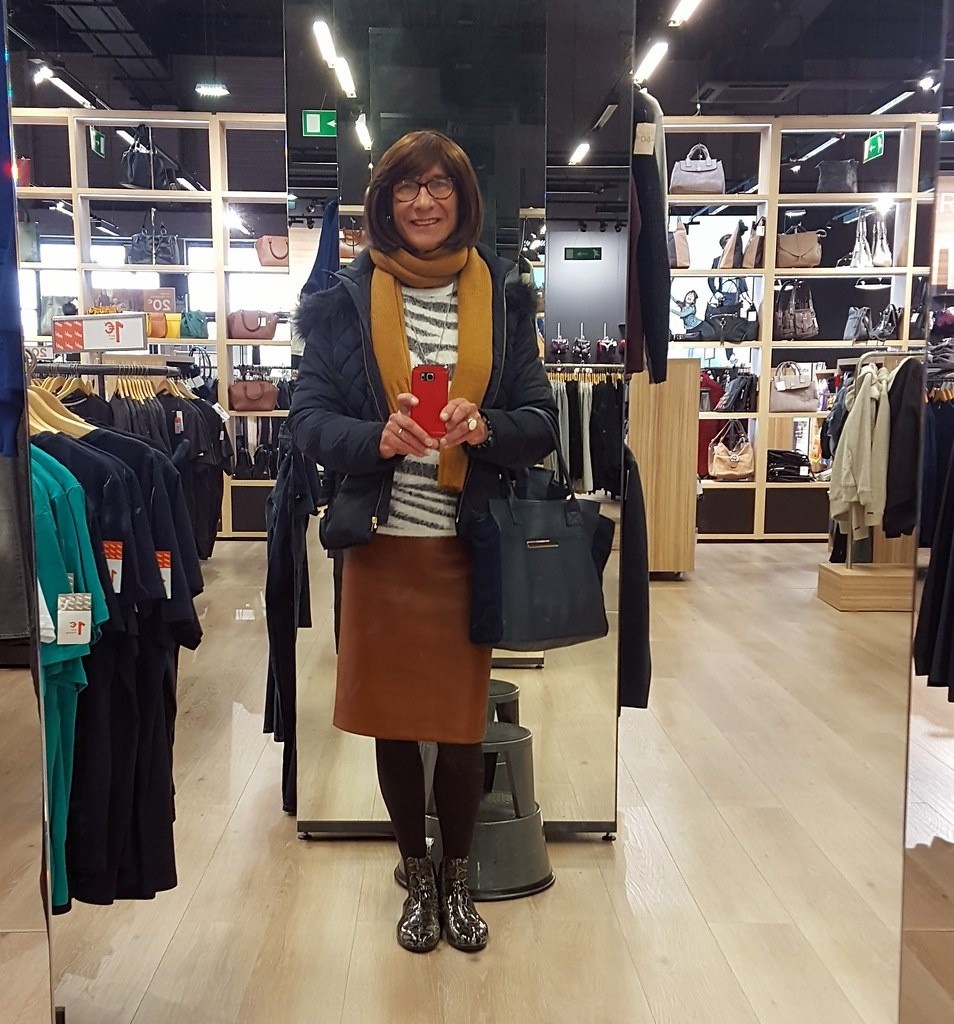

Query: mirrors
[[0, 0, 954, 1024], [280, 0, 740, 851]]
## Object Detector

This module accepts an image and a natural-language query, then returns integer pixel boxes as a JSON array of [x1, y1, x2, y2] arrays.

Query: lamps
[[355, 111, 372, 152], [312, 20, 338, 69], [115, 127, 178, 172], [631, 39, 670, 86], [25, 50, 96, 109], [230, 211, 255, 235], [193, 0, 231, 98], [614, 221, 623, 233], [175, 170, 209, 191], [333, 56, 356, 99], [789, 131, 842, 163], [546, 182, 605, 195], [95, 218, 123, 237], [595, 204, 628, 213], [707, 176, 760, 216], [566, 143, 590, 165], [599, 221, 608, 233], [590, 104, 618, 133], [853, 79, 916, 117], [577, 220, 587, 233], [47, 201, 95, 220], [668, 0, 702, 28]]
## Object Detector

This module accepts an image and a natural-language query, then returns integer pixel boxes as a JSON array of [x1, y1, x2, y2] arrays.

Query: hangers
[[25, 361, 100, 440], [114, 363, 200, 406], [544, 363, 624, 389], [925, 377, 954, 404], [882, 349, 887, 367], [872, 348, 879, 366], [895, 350, 899, 367]]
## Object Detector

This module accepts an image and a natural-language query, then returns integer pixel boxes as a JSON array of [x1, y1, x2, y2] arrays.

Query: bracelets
[[472, 415, 493, 449]]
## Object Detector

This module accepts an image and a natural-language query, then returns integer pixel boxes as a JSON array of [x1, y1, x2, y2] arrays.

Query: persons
[[707, 234, 753, 304], [284, 130, 559, 953], [669, 290, 705, 333]]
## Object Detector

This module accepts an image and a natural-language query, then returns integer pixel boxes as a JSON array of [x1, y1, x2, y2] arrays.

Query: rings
[[399, 428, 403, 434], [466, 418, 477, 431]]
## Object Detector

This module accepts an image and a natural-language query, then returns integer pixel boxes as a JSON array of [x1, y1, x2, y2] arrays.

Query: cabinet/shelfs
[[810, 357, 859, 474], [662, 108, 939, 554], [9, 106, 287, 542]]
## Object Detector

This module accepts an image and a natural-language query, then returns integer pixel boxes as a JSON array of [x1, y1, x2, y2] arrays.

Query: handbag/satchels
[[339, 226, 368, 259], [255, 235, 289, 267], [707, 420, 755, 479], [769, 361, 820, 412], [701, 278, 759, 343], [777, 224, 828, 268], [229, 371, 280, 411], [129, 207, 181, 265], [815, 157, 858, 193], [773, 279, 820, 340], [227, 309, 278, 339], [842, 304, 903, 342], [713, 372, 758, 412], [767, 449, 817, 483], [234, 442, 276, 480], [117, 124, 170, 190], [122, 310, 209, 339], [850, 208, 893, 269], [669, 142, 726, 194], [14, 152, 32, 187], [187, 345, 217, 404], [463, 405, 616, 653], [717, 216, 767, 269], [16, 199, 42, 263]]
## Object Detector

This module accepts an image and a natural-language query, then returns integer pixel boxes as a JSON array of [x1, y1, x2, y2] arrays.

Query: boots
[[395, 856, 442, 953], [436, 856, 488, 953]]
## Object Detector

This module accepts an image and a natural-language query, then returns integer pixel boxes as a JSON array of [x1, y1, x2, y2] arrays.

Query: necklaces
[[404, 284, 455, 365]]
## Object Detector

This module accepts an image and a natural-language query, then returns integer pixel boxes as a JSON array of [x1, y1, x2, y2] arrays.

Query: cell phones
[[410, 366, 450, 439]]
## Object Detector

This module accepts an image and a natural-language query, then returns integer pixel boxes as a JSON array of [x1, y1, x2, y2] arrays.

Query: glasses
[[391, 177, 456, 202]]
